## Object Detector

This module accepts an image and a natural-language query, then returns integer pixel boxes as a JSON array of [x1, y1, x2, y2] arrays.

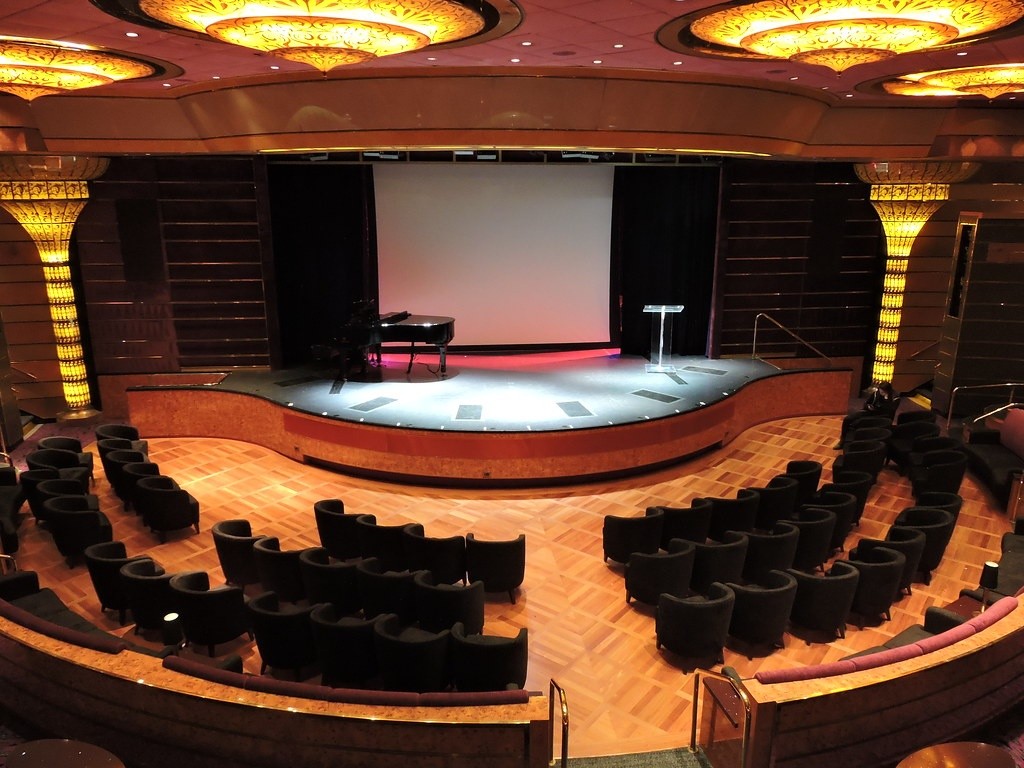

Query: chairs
[[602, 394, 967, 677], [19, 424, 528, 690]]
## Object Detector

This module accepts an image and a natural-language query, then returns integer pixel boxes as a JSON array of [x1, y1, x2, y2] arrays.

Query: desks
[[943, 594, 991, 619], [171, 648, 224, 668]]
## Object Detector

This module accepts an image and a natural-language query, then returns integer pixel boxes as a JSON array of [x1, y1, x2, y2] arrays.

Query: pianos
[[356, 308, 456, 379]]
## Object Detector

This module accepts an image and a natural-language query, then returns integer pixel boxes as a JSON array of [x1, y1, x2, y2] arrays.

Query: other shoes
[[833, 441, 844, 450]]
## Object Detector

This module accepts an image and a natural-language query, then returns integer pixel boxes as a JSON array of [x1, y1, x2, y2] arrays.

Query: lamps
[[871, 183, 949, 389], [164, 613, 184, 656], [0, 180, 93, 410], [654, 0, 1024, 103], [971, 561, 999, 619], [84, 0, 524, 82], [0, 36, 182, 105]]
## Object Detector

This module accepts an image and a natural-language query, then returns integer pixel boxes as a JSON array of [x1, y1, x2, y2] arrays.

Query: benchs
[[0, 452, 551, 768], [688, 520, 1024, 768], [964, 403, 1024, 518]]
